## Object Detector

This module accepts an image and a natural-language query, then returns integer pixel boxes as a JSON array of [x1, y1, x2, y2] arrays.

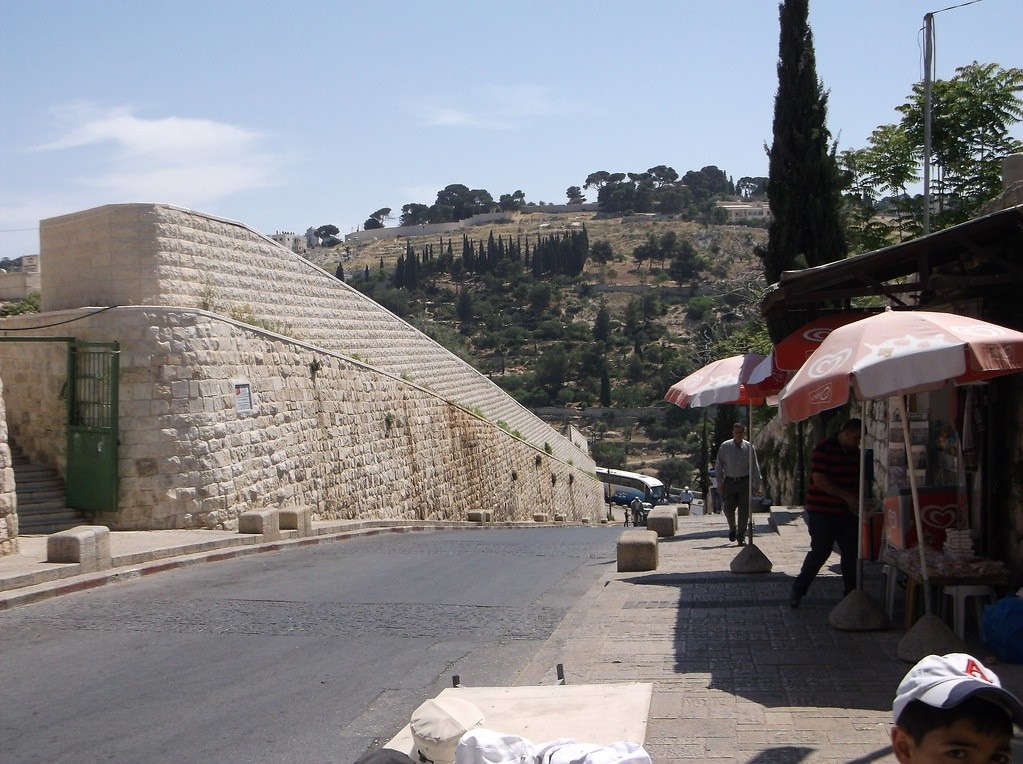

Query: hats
[[893, 653, 1023, 733]]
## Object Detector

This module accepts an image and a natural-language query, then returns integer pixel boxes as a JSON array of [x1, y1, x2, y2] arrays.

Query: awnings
[[757, 202, 1023, 315]]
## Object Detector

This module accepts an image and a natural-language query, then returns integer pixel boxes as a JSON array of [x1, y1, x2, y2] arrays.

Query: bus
[[596, 466, 667, 510]]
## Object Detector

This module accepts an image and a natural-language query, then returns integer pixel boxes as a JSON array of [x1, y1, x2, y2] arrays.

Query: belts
[[725, 475, 749, 480]]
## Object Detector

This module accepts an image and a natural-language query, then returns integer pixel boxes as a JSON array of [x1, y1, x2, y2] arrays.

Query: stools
[[884, 563, 909, 626], [945, 584, 998, 643]]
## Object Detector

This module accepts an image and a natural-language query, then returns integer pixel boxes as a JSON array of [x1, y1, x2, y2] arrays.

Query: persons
[[708, 460, 722, 514], [624, 510, 629, 527], [892, 653, 1023, 764], [715, 423, 762, 545], [679, 486, 693, 511], [631, 497, 644, 528], [789, 418, 867, 607]]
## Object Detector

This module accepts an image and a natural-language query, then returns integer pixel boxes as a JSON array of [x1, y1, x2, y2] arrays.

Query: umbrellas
[[746, 311, 865, 589], [664, 353, 795, 546], [778, 306, 1023, 616]]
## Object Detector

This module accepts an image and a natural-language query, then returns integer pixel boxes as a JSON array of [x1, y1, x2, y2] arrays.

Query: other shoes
[[728, 530, 736, 541], [790, 587, 801, 607], [738, 539, 746, 546]]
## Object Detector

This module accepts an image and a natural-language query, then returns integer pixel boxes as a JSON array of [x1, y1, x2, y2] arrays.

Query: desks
[[882, 550, 1008, 631]]
